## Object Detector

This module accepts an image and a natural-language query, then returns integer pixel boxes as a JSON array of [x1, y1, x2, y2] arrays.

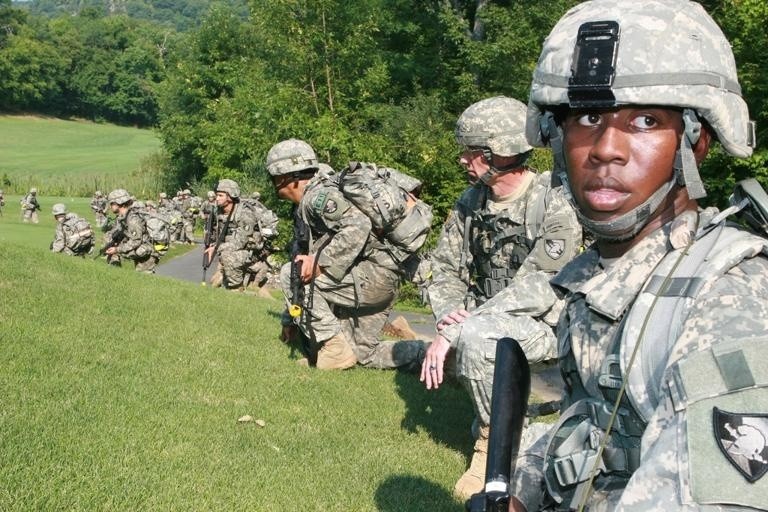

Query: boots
[[452, 423, 491, 505], [293, 330, 357, 371]]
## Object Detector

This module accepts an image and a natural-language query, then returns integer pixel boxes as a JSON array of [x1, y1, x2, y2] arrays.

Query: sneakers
[[173, 238, 195, 246], [381, 316, 416, 341]]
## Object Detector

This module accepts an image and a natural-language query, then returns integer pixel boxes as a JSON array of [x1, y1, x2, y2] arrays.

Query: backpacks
[[246, 199, 281, 238], [324, 161, 435, 266], [21, 194, 29, 211], [135, 211, 171, 258], [62, 214, 94, 256]]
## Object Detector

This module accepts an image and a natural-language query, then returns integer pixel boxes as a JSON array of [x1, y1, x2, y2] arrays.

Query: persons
[[19, 188, 43, 223], [0, 191, 6, 216]]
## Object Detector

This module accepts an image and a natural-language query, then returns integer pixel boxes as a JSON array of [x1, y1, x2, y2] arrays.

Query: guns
[[202, 209, 213, 286], [465, 338, 530, 511], [290, 203, 309, 317], [93, 229, 125, 261]]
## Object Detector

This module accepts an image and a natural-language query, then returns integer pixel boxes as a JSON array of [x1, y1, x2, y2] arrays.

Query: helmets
[[94, 187, 191, 210], [207, 191, 216, 199], [524, 0, 759, 161], [214, 179, 241, 198], [454, 95, 535, 158], [319, 162, 336, 175], [263, 137, 320, 181], [251, 191, 261, 198], [52, 203, 67, 216], [30, 188, 37, 193]]
